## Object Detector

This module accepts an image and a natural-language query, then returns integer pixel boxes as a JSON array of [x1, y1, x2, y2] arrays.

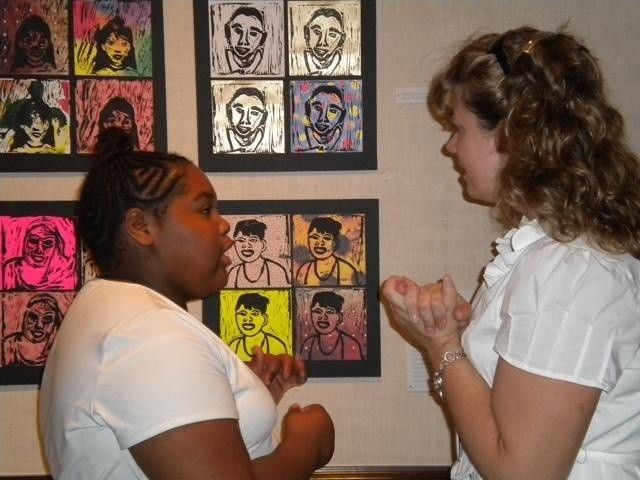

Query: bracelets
[[428, 350, 466, 406]]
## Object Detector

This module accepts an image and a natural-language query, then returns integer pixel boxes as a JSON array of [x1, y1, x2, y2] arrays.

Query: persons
[[378, 26, 640, 480], [39, 127, 334, 480]]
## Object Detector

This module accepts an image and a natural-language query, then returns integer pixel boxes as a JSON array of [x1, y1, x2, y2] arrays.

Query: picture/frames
[[0, 200, 105, 384], [201, 199, 382, 379], [0, 0, 168, 173], [193, 1, 379, 173]]
